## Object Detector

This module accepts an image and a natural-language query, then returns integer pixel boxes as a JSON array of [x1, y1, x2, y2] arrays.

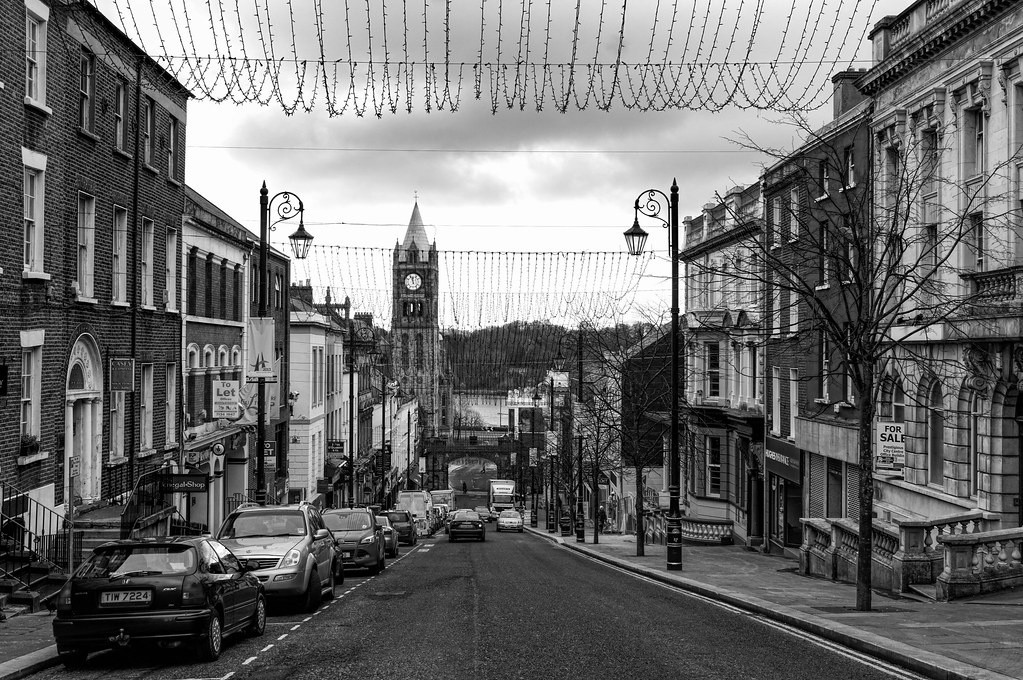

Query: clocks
[[403, 272, 423, 292]]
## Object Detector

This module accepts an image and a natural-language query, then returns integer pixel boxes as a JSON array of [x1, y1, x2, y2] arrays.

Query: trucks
[[396, 489, 433, 536], [486, 479, 516, 519], [429, 489, 456, 511]]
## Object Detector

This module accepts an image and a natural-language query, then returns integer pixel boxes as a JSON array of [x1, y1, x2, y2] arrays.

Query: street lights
[[251, 180, 315, 508], [519, 409, 536, 527], [623, 177, 684, 571], [407, 409, 424, 492], [380, 374, 406, 507], [421, 427, 431, 490], [532, 378, 555, 533], [554, 327, 585, 543], [347, 320, 381, 506]]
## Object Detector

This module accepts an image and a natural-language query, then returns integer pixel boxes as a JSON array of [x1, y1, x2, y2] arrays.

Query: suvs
[[318, 506, 386, 576], [214, 501, 341, 612]]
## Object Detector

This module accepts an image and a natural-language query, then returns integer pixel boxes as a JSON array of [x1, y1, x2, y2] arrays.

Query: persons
[[462, 481, 467, 494], [596, 505, 606, 535]]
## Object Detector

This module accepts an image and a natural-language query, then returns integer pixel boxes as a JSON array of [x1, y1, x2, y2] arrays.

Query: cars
[[50, 536, 269, 666], [433, 503, 492, 544], [495, 510, 523, 533], [380, 509, 418, 546], [374, 515, 399, 559]]
